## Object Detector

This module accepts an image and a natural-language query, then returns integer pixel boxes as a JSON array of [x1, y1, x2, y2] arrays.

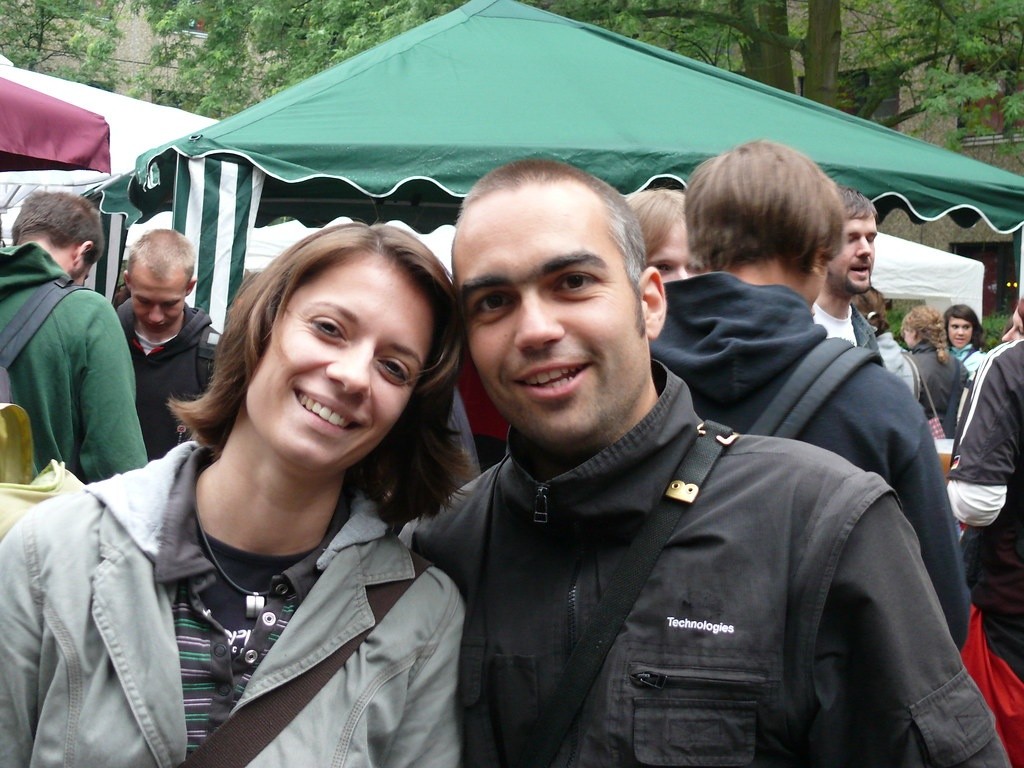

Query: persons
[[940, 305, 988, 390], [810, 184, 890, 375], [397, 157, 1015, 768], [367, 186, 714, 533], [0, 221, 464, 768], [651, 139, 971, 654], [0, 192, 152, 483], [852, 287, 922, 405], [115, 226, 226, 465], [999, 296, 1024, 345], [945, 339, 1024, 680], [900, 305, 966, 440]]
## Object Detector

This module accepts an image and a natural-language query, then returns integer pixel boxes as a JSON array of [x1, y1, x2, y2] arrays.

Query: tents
[[0, 75, 112, 176], [80, 0, 1024, 345]]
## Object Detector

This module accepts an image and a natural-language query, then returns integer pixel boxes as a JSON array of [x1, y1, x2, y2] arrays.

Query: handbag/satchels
[[902, 352, 946, 439]]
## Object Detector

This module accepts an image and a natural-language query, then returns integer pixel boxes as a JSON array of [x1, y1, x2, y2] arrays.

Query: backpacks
[[0, 273, 91, 403]]
